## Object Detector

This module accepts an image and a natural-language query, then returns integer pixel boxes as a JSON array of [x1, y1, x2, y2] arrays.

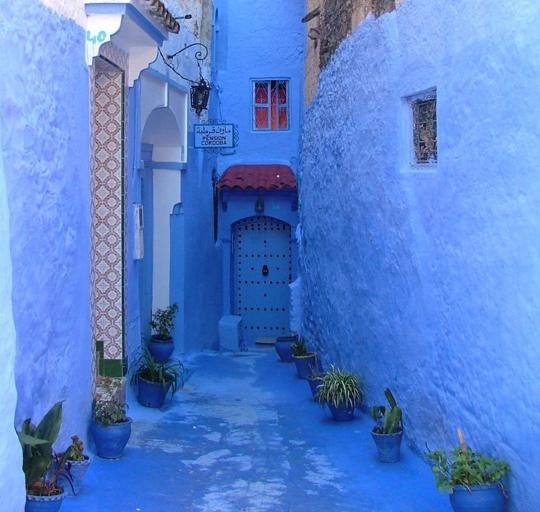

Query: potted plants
[[88, 389, 132, 458], [148, 302, 179, 363], [313, 364, 358, 422], [50, 435, 91, 495], [128, 345, 184, 408], [303, 358, 330, 404], [370, 387, 405, 464], [19, 400, 76, 512], [290, 339, 317, 379], [424, 428, 510, 512]]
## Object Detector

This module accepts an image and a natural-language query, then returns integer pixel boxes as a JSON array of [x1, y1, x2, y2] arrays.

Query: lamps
[[166, 43, 209, 118]]
